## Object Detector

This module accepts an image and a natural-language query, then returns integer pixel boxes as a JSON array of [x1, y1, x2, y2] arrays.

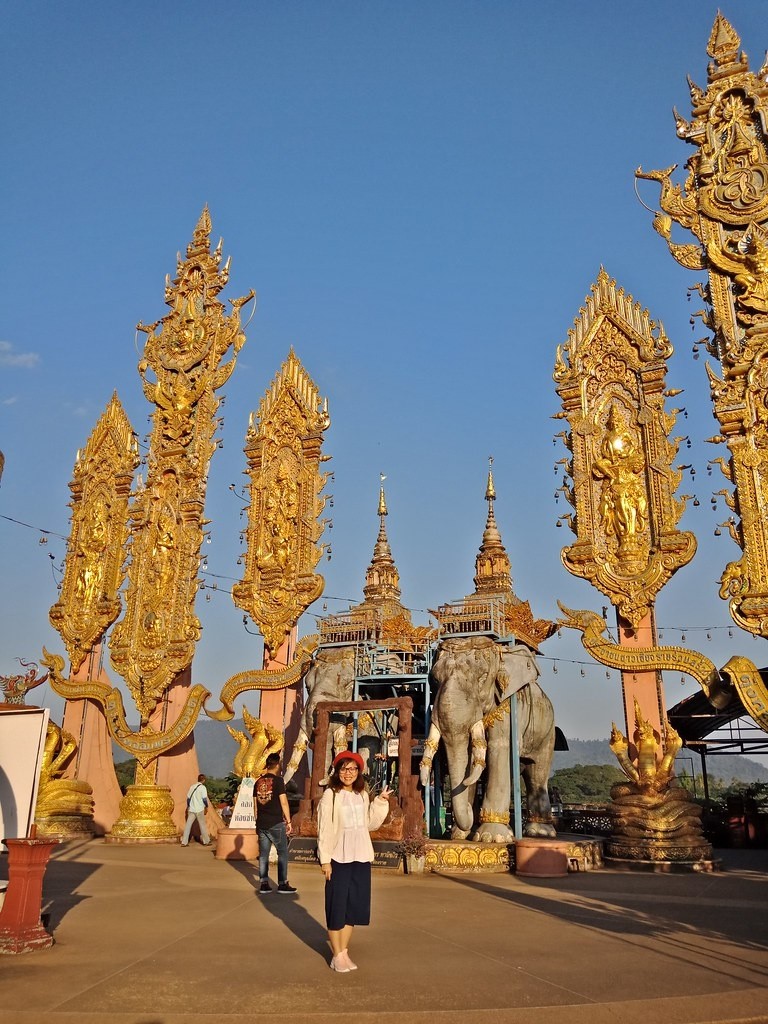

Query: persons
[[181, 774, 213, 847], [253, 752, 298, 894], [222, 804, 234, 817], [317, 751, 394, 972]]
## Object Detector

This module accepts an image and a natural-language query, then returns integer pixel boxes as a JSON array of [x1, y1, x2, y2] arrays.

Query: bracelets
[[286, 821, 291, 824]]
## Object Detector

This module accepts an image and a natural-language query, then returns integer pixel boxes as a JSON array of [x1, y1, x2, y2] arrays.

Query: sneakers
[[330, 949, 357, 972], [277, 881, 297, 893], [260, 883, 273, 893]]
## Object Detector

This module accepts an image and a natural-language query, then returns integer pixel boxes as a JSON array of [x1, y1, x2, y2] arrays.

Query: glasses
[[339, 767, 358, 774]]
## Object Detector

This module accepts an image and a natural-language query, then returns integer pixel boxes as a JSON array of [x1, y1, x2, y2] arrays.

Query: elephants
[[419, 634, 557, 843], [280, 646, 413, 787]]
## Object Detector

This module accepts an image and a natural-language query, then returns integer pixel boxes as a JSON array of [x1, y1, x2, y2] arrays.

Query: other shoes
[[181, 844, 189, 847], [204, 843, 212, 847]]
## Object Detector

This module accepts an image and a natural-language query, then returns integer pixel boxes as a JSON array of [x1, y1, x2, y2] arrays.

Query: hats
[[262, 753, 280, 770], [333, 751, 364, 772]]
